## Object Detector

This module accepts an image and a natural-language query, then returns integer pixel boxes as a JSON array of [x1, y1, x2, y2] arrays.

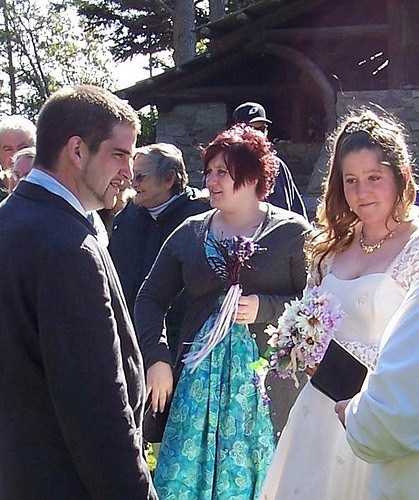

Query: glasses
[[249, 125, 268, 134], [130, 166, 159, 183]]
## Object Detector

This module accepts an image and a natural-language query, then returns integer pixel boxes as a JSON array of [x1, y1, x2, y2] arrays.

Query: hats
[[232, 101, 273, 124]]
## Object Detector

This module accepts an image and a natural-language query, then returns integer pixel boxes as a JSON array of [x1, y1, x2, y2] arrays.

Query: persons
[[201, 102, 309, 222], [108, 142, 216, 425], [0, 115, 36, 202], [334, 272, 419, 500], [258, 106, 419, 500], [0, 85, 159, 499], [132, 123, 316, 500]]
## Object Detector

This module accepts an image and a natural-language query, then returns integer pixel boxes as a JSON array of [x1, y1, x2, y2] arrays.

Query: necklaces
[[359, 221, 401, 254]]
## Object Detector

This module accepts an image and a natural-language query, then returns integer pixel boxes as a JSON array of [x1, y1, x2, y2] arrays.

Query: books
[[309, 337, 370, 402]]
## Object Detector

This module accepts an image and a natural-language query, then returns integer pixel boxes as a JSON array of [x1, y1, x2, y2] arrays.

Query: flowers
[[199, 229, 267, 290], [245, 285, 350, 416]]
